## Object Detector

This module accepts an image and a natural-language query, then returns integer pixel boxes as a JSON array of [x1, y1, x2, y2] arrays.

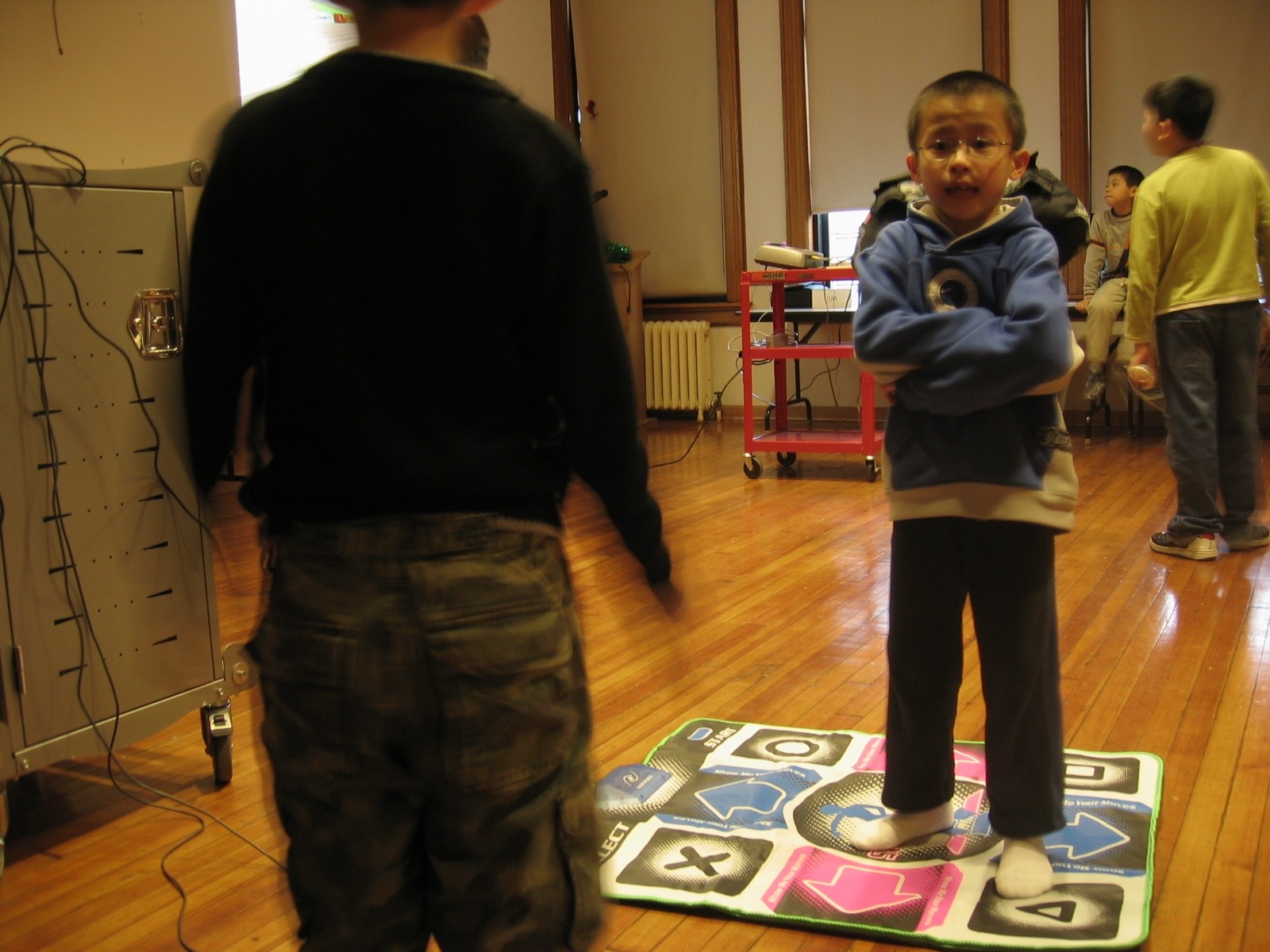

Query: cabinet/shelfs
[[605, 250, 652, 425]]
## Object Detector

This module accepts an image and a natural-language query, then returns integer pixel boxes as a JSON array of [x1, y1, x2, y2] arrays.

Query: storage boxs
[[774, 286, 851, 310]]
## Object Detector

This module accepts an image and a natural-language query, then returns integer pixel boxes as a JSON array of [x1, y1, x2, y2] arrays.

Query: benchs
[[1065, 304, 1158, 438]]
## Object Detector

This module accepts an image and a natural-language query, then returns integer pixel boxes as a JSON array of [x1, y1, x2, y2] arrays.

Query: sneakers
[[1227, 523, 1270, 548], [1149, 529, 1218, 560]]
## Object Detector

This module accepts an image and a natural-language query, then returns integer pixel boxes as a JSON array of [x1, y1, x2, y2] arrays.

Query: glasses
[[914, 129, 1022, 162]]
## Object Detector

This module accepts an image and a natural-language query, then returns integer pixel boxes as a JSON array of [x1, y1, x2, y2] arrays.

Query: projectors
[[754, 244, 824, 269]]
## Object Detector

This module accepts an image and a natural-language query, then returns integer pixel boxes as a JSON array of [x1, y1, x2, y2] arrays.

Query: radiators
[[645, 321, 717, 420]]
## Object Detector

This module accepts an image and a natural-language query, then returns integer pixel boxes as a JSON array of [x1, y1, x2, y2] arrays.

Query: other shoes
[[1083, 365, 1108, 400]]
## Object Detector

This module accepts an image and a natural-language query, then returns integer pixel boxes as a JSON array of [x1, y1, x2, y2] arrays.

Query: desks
[[735, 308, 865, 431]]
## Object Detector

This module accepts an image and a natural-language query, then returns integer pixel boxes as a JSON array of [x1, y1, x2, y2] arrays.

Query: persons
[[1076, 165, 1164, 399], [1122, 77, 1269, 563], [176, 0, 680, 952], [853, 70, 1083, 905], [853, 153, 1089, 269]]
